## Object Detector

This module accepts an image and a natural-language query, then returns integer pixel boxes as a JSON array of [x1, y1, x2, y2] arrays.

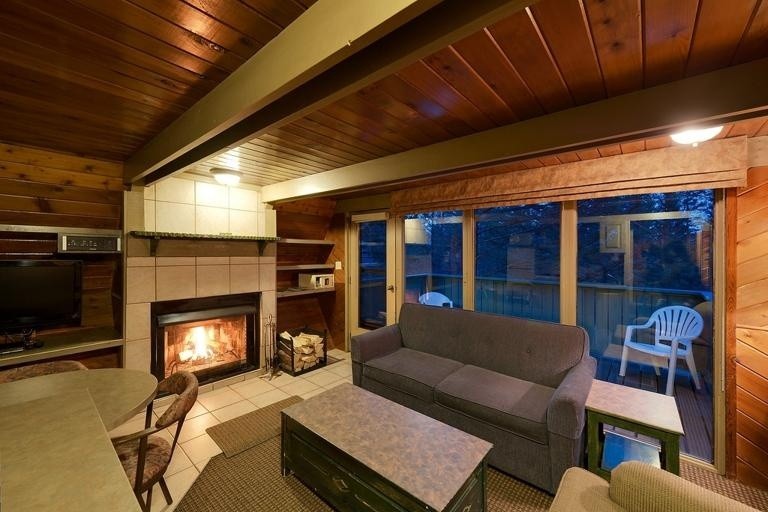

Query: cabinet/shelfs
[[277, 238, 336, 299]]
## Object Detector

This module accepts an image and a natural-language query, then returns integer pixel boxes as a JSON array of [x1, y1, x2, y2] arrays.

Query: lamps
[[671, 126, 724, 144], [209, 168, 242, 186]]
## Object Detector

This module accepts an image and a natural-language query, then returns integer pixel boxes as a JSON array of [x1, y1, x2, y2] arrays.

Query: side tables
[[603, 343, 669, 370], [585, 378, 685, 484]]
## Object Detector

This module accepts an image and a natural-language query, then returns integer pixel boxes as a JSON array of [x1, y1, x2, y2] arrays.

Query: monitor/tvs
[[0, 260, 83, 333]]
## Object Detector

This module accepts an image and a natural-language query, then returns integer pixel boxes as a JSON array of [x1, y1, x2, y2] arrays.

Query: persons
[[648, 240, 708, 309]]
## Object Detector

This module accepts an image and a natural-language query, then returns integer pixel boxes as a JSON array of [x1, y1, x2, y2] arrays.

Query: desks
[[0, 387, 160, 512], [0, 369, 158, 432], [0, 325, 123, 368]]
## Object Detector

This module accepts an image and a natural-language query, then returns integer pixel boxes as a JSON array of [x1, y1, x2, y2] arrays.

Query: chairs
[[110, 370, 198, 512], [0, 362, 88, 384], [549, 460, 768, 512], [616, 301, 713, 397], [419, 292, 453, 308]]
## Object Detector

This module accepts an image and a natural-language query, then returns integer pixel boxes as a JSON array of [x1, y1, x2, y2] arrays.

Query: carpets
[[174, 454, 768, 512], [205, 394, 304, 458]]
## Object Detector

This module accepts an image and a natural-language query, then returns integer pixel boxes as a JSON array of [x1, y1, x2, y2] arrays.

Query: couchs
[[351, 303, 598, 496]]
